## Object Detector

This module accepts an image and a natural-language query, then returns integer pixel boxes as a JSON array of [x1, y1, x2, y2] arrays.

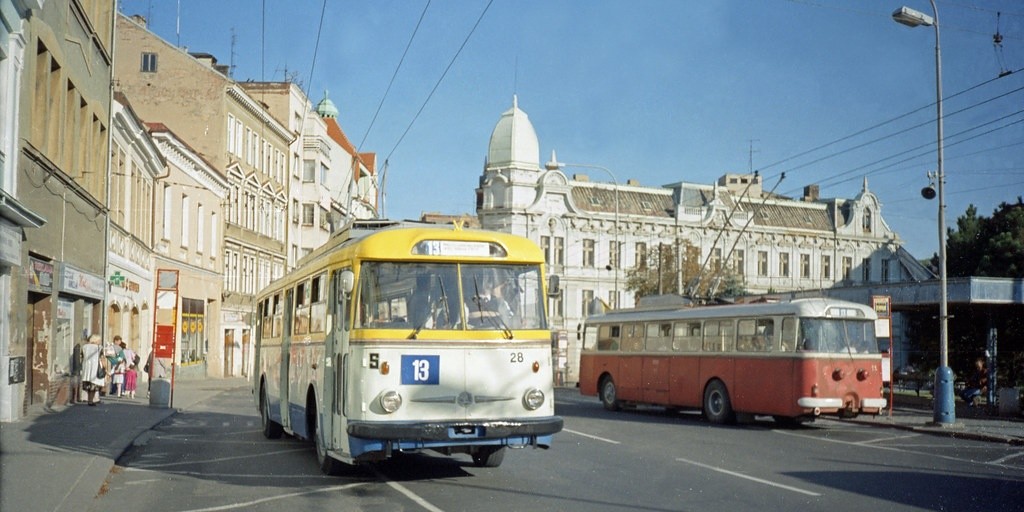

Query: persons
[[455, 272, 514, 331], [960, 358, 993, 409], [407, 264, 433, 326], [80, 334, 154, 406]]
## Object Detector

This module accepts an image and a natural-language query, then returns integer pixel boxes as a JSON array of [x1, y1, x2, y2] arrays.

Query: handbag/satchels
[[97, 366, 107, 379]]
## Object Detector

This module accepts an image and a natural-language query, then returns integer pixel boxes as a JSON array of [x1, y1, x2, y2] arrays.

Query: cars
[[893, 364, 921, 382]]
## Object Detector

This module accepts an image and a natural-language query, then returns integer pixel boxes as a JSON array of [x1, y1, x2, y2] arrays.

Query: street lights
[[892, 0, 956, 421], [545, 161, 619, 308]]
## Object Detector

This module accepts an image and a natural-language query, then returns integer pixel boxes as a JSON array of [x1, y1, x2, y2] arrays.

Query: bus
[[578, 293, 888, 427], [254, 220, 564, 474]]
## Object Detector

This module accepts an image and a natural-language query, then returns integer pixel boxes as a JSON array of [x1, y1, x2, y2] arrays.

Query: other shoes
[[88, 403, 96, 406]]
